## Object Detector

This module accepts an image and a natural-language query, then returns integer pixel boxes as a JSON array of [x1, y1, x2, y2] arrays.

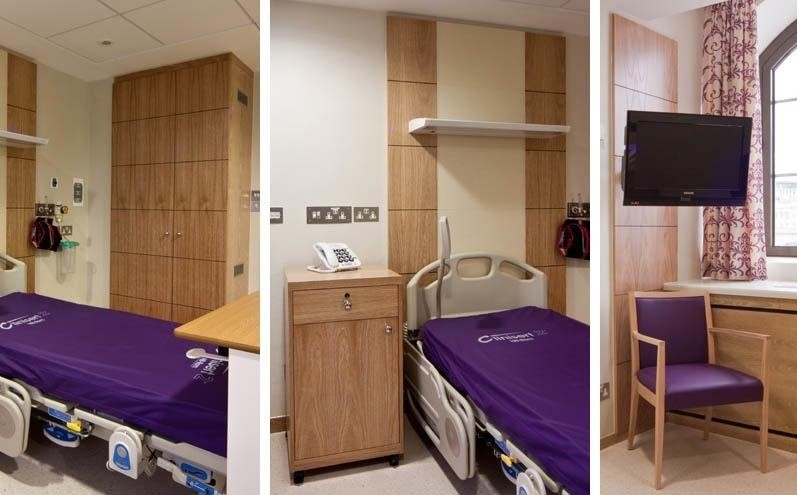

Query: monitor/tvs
[[622, 109, 753, 206]]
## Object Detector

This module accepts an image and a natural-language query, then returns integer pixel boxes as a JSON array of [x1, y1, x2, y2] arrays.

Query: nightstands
[[282, 262, 405, 485]]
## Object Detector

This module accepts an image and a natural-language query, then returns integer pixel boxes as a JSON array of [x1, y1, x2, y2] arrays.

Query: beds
[[0, 254, 227, 495], [405, 251, 589, 493]]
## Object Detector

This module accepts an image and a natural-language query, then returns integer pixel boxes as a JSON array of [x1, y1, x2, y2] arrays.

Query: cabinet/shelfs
[[109, 52, 254, 324]]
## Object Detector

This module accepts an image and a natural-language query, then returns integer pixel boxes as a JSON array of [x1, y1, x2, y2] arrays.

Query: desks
[[174, 291, 259, 495]]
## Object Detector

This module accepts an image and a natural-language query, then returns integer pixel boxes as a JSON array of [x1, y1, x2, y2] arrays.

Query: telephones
[[312, 242, 361, 271]]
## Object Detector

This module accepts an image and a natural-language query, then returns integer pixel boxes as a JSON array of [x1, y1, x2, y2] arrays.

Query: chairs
[[626, 289, 771, 491]]
